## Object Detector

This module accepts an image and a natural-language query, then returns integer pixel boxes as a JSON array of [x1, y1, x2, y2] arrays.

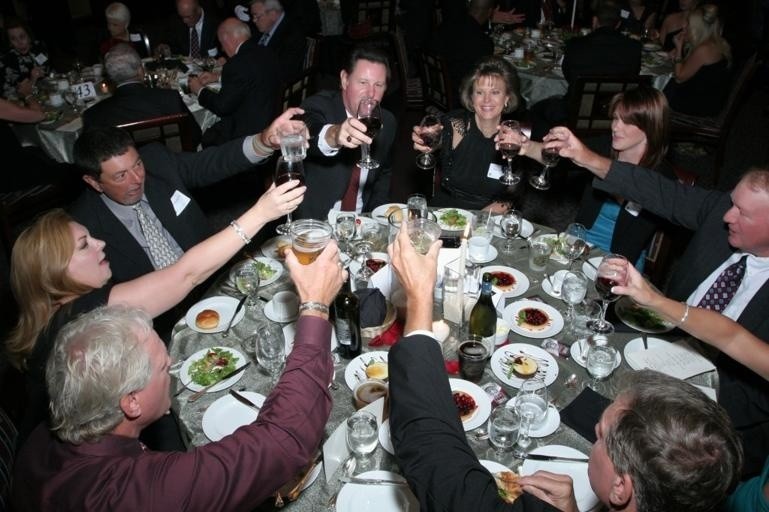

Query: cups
[[139, 42, 225, 101], [35, 65, 113, 128], [277, 124, 308, 162]]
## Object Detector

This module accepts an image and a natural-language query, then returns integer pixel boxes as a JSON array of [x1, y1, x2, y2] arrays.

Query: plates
[[638, 24, 670, 77], [491, 17, 591, 76]]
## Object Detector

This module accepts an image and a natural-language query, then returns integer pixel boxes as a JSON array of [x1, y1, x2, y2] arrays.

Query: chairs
[[271, 68, 314, 119], [575, 75, 653, 145], [115, 112, 196, 150], [415, 60, 454, 116]]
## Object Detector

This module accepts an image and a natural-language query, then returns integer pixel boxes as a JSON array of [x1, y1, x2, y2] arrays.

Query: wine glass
[[415, 115, 442, 170], [354, 98, 385, 171], [274, 154, 309, 235], [496, 120, 524, 187], [174, 194, 721, 511], [528, 120, 563, 189]]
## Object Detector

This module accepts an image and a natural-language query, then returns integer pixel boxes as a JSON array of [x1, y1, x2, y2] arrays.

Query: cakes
[[513, 357, 538, 378]]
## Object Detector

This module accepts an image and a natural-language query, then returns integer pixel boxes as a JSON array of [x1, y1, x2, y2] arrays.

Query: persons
[[540, 127, 769, 428], [2, 179, 307, 463], [593, 256, 769, 512], [7, 238, 348, 512], [378, 230, 742, 512], [0, 0, 769, 264]]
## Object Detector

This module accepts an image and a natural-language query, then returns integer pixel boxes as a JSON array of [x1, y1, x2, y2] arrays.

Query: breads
[[197, 310, 219, 329], [384, 206, 404, 224]]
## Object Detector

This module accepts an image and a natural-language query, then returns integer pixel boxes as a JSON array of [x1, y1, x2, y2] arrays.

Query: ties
[[341, 147, 361, 211], [191, 24, 201, 60], [132, 201, 178, 270], [696, 255, 748, 313]]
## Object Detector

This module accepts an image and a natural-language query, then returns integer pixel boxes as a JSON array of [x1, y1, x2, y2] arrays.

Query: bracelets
[[299, 302, 329, 316], [677, 303, 689, 326]]
